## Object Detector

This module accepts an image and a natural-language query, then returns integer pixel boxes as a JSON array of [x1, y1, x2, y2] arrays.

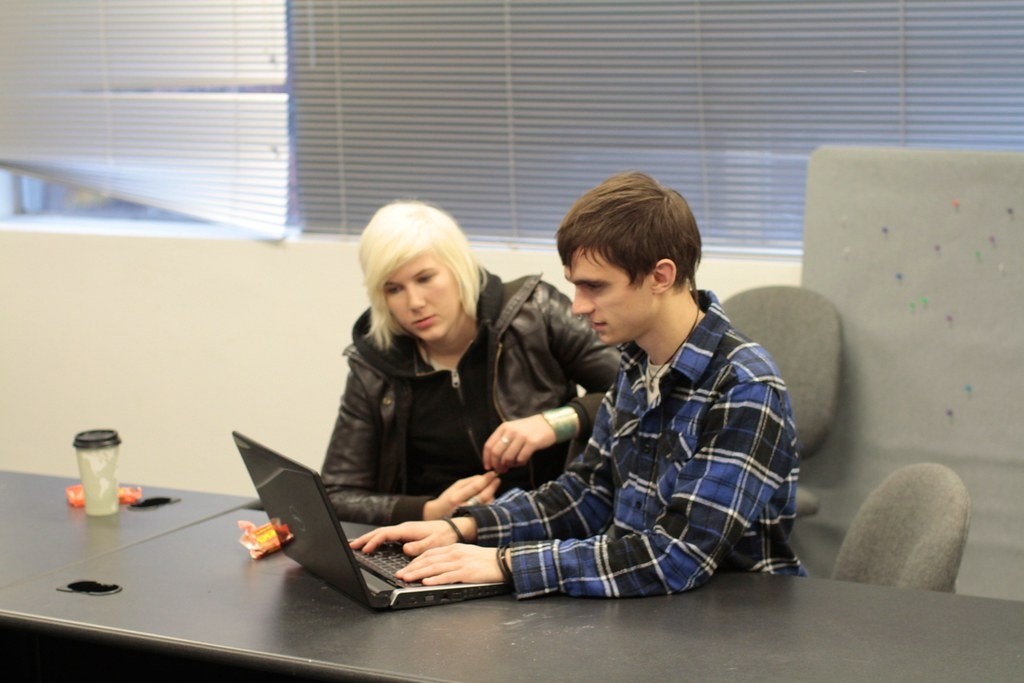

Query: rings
[[469, 498, 480, 506], [501, 437, 509, 446]]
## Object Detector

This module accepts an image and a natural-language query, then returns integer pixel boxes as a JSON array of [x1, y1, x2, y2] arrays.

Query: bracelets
[[443, 516, 466, 542], [496, 544, 514, 582], [543, 408, 576, 442]]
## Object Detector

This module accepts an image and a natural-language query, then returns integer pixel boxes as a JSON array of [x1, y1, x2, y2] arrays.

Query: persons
[[348, 173, 807, 600], [319, 199, 624, 524]]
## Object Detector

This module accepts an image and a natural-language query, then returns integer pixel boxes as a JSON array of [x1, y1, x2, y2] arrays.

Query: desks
[[0, 507, 1024, 683], [0, 470, 260, 590]]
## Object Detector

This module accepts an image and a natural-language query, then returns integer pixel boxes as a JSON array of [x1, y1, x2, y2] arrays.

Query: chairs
[[830, 462, 973, 593], [721, 284, 845, 519]]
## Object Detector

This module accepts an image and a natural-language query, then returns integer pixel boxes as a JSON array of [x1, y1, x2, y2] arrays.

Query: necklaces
[[648, 301, 700, 393]]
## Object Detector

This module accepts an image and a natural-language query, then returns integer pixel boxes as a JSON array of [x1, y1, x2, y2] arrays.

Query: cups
[[73, 430, 121, 516]]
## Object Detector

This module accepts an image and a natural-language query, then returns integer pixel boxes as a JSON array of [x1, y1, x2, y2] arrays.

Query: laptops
[[232, 432, 512, 610]]
